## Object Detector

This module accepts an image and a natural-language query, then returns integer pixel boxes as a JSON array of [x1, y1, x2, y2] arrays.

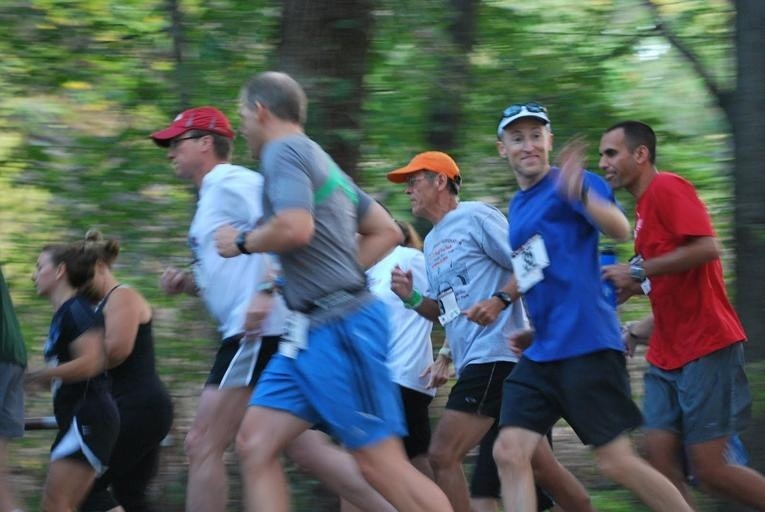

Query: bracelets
[[629, 331, 638, 340], [404, 289, 423, 309]]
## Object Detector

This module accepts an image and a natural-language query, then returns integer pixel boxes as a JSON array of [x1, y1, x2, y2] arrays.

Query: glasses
[[496, 103, 550, 117], [408, 173, 437, 186], [168, 135, 204, 146]]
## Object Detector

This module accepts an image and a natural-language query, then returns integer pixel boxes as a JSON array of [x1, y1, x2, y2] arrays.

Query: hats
[[496, 106, 550, 129], [387, 151, 461, 184], [151, 106, 233, 149]]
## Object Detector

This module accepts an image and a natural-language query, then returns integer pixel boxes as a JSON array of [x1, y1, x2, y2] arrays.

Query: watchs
[[233, 233, 251, 258], [493, 292, 512, 310], [628, 265, 645, 284]]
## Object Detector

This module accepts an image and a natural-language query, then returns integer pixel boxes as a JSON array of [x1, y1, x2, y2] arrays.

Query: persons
[[623, 313, 750, 484], [492, 101, 692, 508], [215, 71, 450, 511], [600, 120, 765, 511], [2, 273, 29, 442], [24, 246, 119, 511], [152, 109, 386, 511], [79, 241, 174, 505], [474, 412, 555, 512], [342, 193, 456, 512], [385, 149, 589, 511]]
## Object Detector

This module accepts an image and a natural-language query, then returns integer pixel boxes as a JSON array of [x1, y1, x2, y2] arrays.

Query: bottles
[[600, 243, 617, 314]]
